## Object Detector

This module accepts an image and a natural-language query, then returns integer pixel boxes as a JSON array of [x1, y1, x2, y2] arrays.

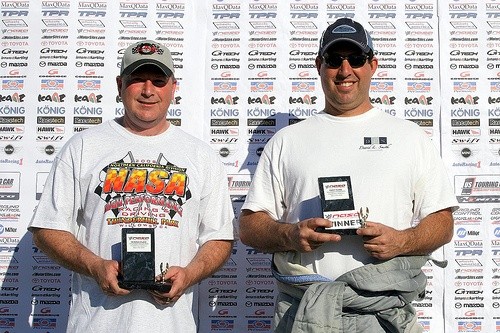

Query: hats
[[120, 40, 175, 80], [317, 18, 374, 59]]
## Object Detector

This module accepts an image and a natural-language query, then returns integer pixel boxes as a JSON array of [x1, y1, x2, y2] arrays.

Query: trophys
[[356, 206, 371, 240], [153, 262, 173, 294]]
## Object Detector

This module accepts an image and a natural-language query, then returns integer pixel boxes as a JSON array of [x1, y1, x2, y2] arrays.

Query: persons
[[27, 41, 237, 333], [236, 17, 460, 333]]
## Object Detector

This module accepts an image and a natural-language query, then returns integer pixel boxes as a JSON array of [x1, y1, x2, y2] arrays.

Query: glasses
[[319, 53, 371, 68]]
